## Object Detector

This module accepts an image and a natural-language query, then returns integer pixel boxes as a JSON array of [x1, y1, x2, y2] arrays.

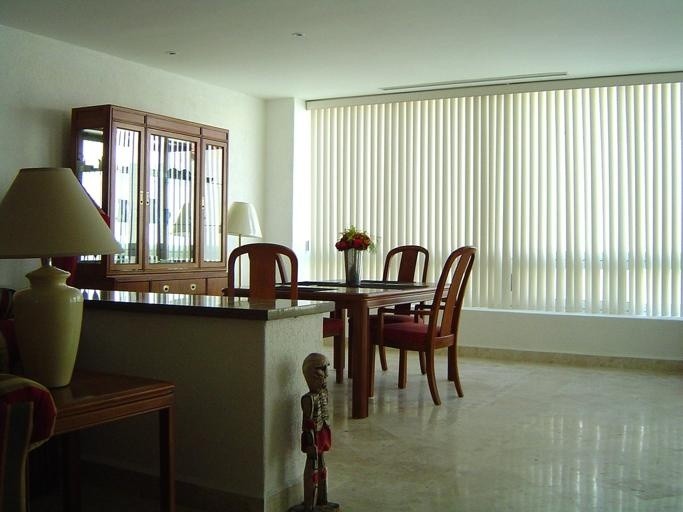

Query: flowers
[[335, 225, 377, 255]]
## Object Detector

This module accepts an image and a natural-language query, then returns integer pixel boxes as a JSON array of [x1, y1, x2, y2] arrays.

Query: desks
[[221, 282, 461, 419]]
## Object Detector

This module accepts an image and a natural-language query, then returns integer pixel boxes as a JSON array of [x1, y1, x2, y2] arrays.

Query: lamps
[[0, 167, 125, 388], [218, 202, 262, 297]]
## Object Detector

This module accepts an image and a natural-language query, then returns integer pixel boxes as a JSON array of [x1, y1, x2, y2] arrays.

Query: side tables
[[11, 368, 177, 511]]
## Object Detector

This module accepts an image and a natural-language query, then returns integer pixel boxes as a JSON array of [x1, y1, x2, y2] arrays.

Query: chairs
[[370, 246, 476, 405], [228, 243, 345, 384], [368, 246, 426, 374]]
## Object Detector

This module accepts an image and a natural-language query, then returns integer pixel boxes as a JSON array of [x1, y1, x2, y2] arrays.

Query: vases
[[344, 248, 362, 287]]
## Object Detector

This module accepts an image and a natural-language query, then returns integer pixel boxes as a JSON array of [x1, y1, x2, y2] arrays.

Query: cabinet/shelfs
[[69, 104, 229, 297]]
